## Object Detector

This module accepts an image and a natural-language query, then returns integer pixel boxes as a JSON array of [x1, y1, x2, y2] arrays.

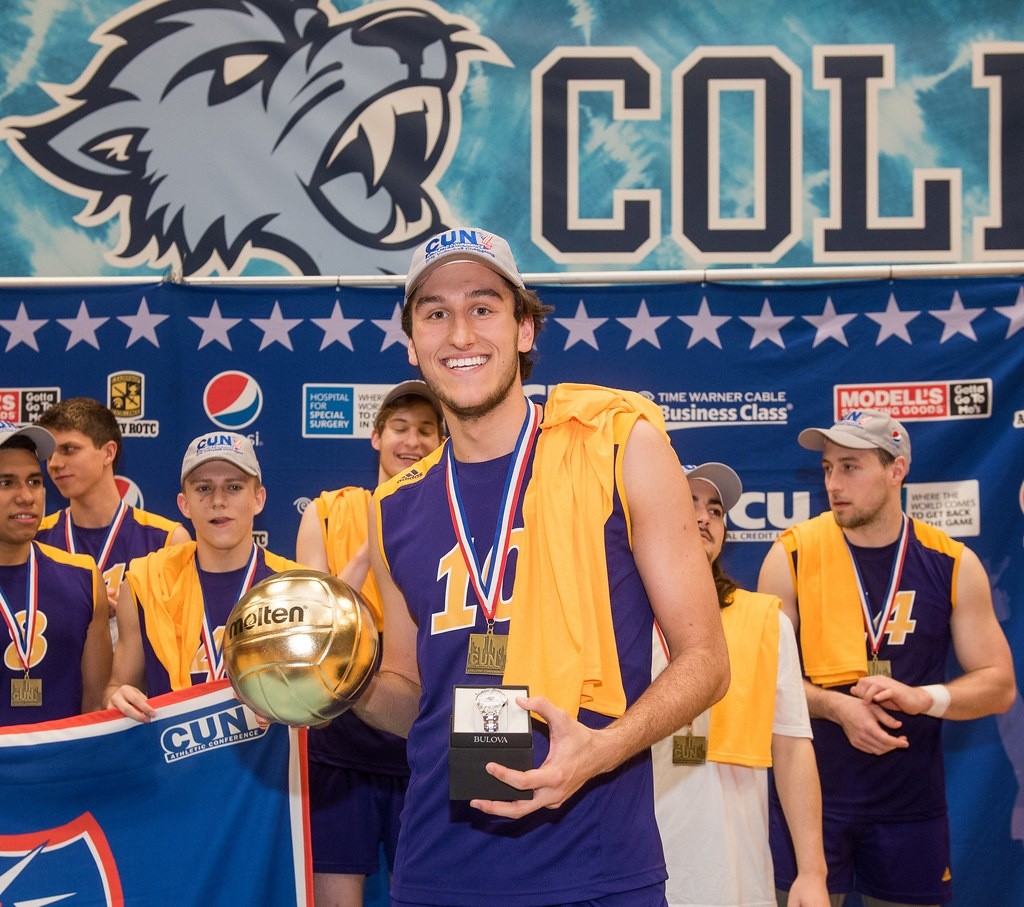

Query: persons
[[650, 461, 830, 907], [103, 432, 319, 722], [297, 379, 446, 906], [758, 409, 1016, 907], [34, 399, 193, 658], [235, 226, 730, 907], [1, 421, 113, 729]]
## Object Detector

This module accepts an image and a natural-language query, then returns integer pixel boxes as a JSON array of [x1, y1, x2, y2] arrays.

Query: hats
[[377, 381, 440, 409], [2, 427, 56, 460], [402, 229, 525, 300], [183, 430, 266, 479], [683, 461, 742, 514], [793, 408, 914, 461]]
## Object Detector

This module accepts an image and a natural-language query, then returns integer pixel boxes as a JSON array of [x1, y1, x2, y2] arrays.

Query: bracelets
[[919, 684, 950, 717]]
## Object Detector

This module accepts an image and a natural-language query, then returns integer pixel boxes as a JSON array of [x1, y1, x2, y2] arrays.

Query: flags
[[0, 678, 315, 907]]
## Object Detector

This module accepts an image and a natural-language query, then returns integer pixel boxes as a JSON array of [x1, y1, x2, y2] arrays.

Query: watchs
[[476, 689, 507, 733]]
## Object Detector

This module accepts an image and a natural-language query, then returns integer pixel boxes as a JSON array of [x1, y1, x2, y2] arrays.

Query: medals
[[863, 660, 891, 678], [466, 634, 507, 675], [673, 735, 706, 764], [11, 679, 42, 706]]
